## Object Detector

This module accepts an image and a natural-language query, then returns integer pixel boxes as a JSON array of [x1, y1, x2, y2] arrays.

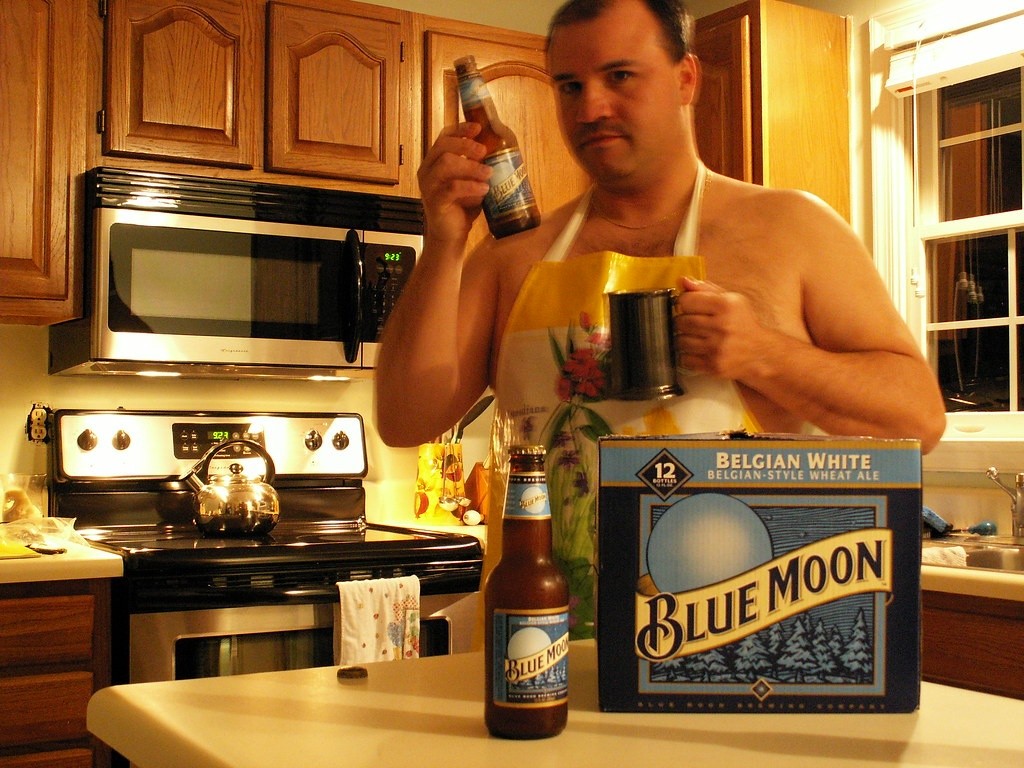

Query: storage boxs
[[595, 430, 923, 714]]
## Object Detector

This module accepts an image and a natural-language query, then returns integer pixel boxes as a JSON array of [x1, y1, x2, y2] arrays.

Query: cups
[[0, 472, 46, 522], [412, 443, 466, 527], [604, 286, 687, 401]]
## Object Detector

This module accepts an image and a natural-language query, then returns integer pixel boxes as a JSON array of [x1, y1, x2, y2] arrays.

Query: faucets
[[985, 465, 1024, 537]]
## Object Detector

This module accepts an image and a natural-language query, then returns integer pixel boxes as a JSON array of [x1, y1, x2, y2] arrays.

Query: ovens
[[128, 589, 482, 690]]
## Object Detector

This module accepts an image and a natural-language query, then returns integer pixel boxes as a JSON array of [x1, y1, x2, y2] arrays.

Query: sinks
[[922, 548, 1024, 575]]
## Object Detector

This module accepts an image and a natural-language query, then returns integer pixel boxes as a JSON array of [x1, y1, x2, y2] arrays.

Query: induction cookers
[[48, 405, 481, 605]]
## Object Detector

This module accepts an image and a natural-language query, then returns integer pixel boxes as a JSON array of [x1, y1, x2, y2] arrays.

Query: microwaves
[[47, 166, 430, 381]]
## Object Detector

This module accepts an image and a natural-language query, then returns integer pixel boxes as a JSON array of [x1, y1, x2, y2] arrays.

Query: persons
[[372, 0, 947, 638]]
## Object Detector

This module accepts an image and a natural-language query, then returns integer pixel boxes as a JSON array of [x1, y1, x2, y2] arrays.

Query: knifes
[[482, 446, 496, 469]]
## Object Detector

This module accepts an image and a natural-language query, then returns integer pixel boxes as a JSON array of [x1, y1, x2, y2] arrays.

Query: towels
[[333, 574, 421, 666], [922, 545, 968, 568]]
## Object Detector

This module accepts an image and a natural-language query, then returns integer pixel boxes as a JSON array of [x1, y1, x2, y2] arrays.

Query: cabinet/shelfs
[[693, 0, 853, 226], [0, 533, 123, 768], [0, 0, 88, 327], [414, 11, 590, 256], [921, 564, 1024, 701], [88, 0, 414, 204]]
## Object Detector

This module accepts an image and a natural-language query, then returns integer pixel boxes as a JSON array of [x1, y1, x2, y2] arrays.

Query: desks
[[85, 637, 1024, 768]]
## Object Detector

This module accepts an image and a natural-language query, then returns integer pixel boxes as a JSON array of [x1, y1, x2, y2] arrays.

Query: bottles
[[482, 444, 571, 740], [452, 53, 541, 239]]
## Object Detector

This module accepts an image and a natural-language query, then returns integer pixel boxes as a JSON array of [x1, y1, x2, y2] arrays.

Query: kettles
[[178, 438, 282, 534]]
